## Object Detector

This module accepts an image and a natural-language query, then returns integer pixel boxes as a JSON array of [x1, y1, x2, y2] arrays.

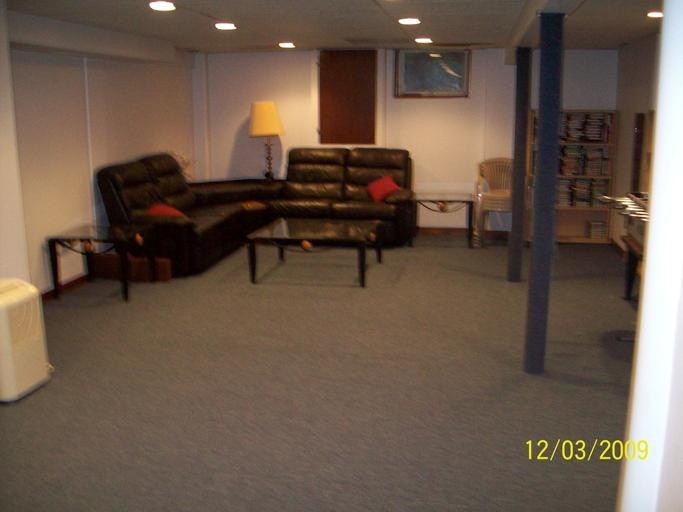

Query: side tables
[[47, 222, 154, 304], [408, 190, 474, 247]]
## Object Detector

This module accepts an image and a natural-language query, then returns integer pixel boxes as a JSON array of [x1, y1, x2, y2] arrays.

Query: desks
[[618, 234, 642, 300]]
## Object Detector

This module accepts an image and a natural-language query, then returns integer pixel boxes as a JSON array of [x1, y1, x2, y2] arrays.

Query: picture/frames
[[393, 48, 471, 99]]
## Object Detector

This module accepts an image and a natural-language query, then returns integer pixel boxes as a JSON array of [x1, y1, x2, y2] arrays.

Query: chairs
[[472, 158, 513, 249]]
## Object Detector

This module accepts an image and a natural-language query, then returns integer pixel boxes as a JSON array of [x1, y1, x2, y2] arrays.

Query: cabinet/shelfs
[[521, 108, 616, 243]]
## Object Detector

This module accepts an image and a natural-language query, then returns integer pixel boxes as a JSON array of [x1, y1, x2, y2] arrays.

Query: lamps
[[248, 100, 284, 182]]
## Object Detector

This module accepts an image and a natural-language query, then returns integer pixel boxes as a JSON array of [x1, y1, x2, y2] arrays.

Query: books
[[558, 112, 611, 207], [585, 219, 606, 240]]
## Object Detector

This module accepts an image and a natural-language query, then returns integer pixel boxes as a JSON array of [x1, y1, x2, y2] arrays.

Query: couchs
[[269, 147, 420, 243], [95, 150, 282, 279]]
